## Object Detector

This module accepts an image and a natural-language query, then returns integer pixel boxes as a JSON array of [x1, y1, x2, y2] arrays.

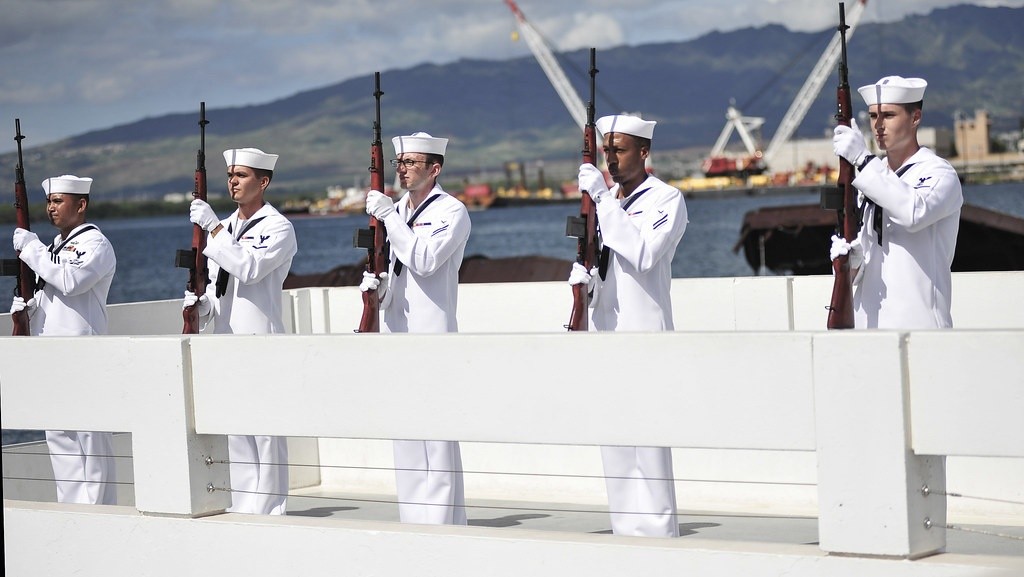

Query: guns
[[820, 2, 864, 330], [352, 72, 391, 333], [174, 102, 212, 334], [564, 48, 600, 331], [0, 118, 35, 336]]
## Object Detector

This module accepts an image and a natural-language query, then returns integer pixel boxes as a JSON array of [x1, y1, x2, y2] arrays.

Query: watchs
[[858, 155, 876, 172]]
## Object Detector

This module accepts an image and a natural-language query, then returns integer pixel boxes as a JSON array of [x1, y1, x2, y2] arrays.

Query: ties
[[599, 188, 650, 282], [393, 194, 440, 277], [216, 217, 265, 298], [34, 226, 95, 294], [873, 164, 914, 246]]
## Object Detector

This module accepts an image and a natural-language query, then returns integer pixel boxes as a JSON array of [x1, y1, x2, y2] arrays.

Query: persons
[[359, 132, 472, 524], [830, 74, 965, 331], [10, 174, 118, 506], [183, 147, 298, 517], [568, 114, 688, 537]]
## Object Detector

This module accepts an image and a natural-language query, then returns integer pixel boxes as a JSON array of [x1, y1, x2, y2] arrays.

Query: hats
[[857, 75, 928, 106], [596, 115, 657, 139], [223, 148, 278, 170], [392, 132, 448, 155]]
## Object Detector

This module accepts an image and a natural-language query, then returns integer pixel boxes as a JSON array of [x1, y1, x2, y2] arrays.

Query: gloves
[[190, 199, 221, 233], [13, 228, 39, 251], [833, 118, 871, 166], [366, 190, 395, 221], [182, 291, 210, 317], [569, 262, 598, 294], [830, 235, 862, 265], [42, 175, 93, 194], [10, 296, 37, 319], [359, 272, 389, 300], [578, 163, 608, 202]]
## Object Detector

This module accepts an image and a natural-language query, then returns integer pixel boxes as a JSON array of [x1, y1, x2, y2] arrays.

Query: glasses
[[390, 159, 434, 170]]
